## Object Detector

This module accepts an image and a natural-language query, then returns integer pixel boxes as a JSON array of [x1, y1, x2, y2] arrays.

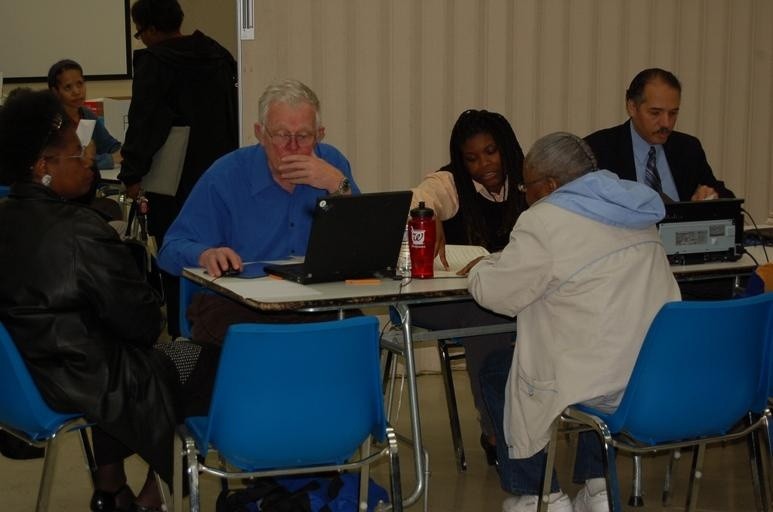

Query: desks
[[181, 243, 773, 472], [98, 167, 138, 239]]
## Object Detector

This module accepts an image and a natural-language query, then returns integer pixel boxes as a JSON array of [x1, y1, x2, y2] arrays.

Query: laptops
[[262, 189, 413, 285]]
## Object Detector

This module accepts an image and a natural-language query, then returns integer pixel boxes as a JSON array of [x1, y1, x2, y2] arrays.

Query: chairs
[[385, 306, 479, 473], [172, 315, 404, 512], [0, 321, 97, 512], [537, 293, 773, 512]]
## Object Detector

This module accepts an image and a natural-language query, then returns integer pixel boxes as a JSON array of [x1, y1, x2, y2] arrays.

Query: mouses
[[218, 257, 243, 277]]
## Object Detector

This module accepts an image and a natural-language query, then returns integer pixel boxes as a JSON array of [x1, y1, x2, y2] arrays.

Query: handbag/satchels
[[217, 471, 388, 512], [124, 238, 166, 305]]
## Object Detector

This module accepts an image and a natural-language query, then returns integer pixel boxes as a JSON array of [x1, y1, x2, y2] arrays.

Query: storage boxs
[[83, 96, 131, 145]]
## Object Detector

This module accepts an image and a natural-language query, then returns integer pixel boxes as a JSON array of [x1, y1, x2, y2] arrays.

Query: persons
[[121, 0, 237, 342], [0, 86, 222, 512], [456, 131, 683, 512], [583, 68, 737, 201], [48, 60, 126, 169], [156, 80, 363, 416], [397, 110, 529, 465]]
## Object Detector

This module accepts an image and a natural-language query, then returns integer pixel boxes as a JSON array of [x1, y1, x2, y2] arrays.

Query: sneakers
[[573, 481, 609, 512], [502, 491, 573, 512]]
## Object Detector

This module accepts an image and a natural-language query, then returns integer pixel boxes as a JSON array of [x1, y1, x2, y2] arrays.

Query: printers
[[655, 196, 748, 266]]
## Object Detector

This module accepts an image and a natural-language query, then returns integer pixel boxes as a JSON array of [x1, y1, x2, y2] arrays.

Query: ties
[[643, 147, 662, 193]]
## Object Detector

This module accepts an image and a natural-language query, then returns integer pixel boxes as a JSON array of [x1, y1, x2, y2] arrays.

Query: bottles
[[407, 201, 437, 279]]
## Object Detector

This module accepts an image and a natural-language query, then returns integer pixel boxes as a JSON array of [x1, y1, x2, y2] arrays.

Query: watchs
[[330, 176, 351, 197]]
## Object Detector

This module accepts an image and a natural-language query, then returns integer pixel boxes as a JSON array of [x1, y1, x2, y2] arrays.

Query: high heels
[[480, 434, 497, 465]]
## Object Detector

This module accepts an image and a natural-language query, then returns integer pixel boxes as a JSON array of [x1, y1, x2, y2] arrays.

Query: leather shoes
[[129, 501, 160, 512], [91, 484, 133, 512]]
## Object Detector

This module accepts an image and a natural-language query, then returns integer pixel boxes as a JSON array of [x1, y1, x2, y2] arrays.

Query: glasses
[[133, 26, 146, 40], [518, 175, 557, 191], [49, 146, 87, 162], [262, 124, 316, 148]]
